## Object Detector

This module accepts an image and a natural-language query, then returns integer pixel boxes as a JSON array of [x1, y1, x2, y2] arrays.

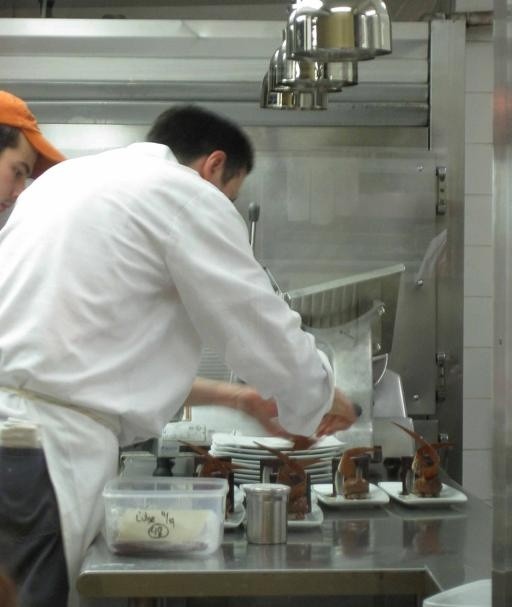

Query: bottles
[[154, 458, 173, 478], [244, 482, 292, 545]]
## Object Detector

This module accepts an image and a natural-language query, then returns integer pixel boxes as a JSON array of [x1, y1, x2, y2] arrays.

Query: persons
[[0, 90, 64, 225], [0, 101, 357, 607]]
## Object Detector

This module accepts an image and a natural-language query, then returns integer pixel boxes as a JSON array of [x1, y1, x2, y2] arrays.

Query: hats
[[0, 90, 66, 179]]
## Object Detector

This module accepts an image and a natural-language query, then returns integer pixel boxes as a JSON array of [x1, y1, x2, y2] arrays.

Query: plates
[[202, 432, 468, 531]]
